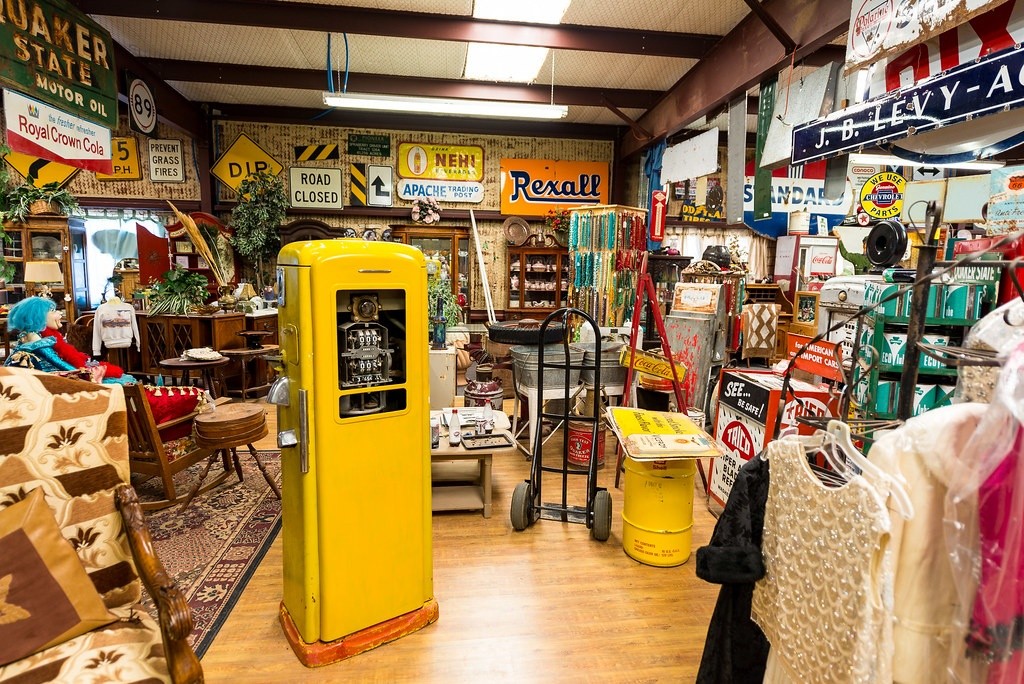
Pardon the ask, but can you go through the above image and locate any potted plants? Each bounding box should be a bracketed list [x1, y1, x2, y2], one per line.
[0, 176, 82, 280]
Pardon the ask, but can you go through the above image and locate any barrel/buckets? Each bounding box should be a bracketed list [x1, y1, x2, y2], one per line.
[564, 420, 606, 471]
[622, 454, 697, 567]
[788, 210, 810, 235]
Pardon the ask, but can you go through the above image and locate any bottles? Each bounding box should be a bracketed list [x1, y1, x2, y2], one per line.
[430, 417, 439, 449]
[129, 276, 162, 311]
[433, 297, 446, 343]
[217, 273, 273, 312]
[484, 399, 494, 433]
[535, 224, 547, 248]
[449, 409, 461, 447]
[510, 260, 570, 307]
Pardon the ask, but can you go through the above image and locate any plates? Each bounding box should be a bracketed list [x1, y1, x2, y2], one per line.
[503, 216, 531, 245]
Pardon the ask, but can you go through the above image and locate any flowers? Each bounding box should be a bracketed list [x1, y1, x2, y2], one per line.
[545, 209, 571, 233]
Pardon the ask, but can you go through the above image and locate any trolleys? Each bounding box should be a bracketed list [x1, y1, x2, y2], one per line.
[510, 306, 612, 540]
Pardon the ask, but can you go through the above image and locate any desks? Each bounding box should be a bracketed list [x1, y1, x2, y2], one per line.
[512, 384, 620, 459]
[429, 427, 492, 519]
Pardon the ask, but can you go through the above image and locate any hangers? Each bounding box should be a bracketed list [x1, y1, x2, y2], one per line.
[796, 386, 914, 518]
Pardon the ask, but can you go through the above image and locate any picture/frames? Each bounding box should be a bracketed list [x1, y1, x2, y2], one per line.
[793, 291, 821, 325]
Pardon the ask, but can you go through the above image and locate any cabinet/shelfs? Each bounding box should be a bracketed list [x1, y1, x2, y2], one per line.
[246, 319, 279, 386]
[505, 245, 569, 321]
[120, 316, 246, 401]
[844, 309, 990, 460]
[0, 218, 76, 334]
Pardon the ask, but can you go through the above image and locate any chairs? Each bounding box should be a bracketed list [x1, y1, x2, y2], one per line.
[0, 363, 204, 684]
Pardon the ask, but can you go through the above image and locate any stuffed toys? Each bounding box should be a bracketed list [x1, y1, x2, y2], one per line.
[8, 297, 163, 386]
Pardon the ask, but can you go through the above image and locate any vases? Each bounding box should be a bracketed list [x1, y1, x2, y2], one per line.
[555, 230, 569, 247]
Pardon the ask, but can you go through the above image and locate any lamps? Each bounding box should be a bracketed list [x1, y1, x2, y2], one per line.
[322, 34, 568, 120]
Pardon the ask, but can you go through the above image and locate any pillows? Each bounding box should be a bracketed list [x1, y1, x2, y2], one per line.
[0, 488, 122, 667]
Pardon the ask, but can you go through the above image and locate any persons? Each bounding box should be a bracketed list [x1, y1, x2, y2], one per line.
[833, 230, 874, 274]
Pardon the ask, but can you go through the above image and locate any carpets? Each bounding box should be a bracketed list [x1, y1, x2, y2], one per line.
[153, 449, 285, 658]
